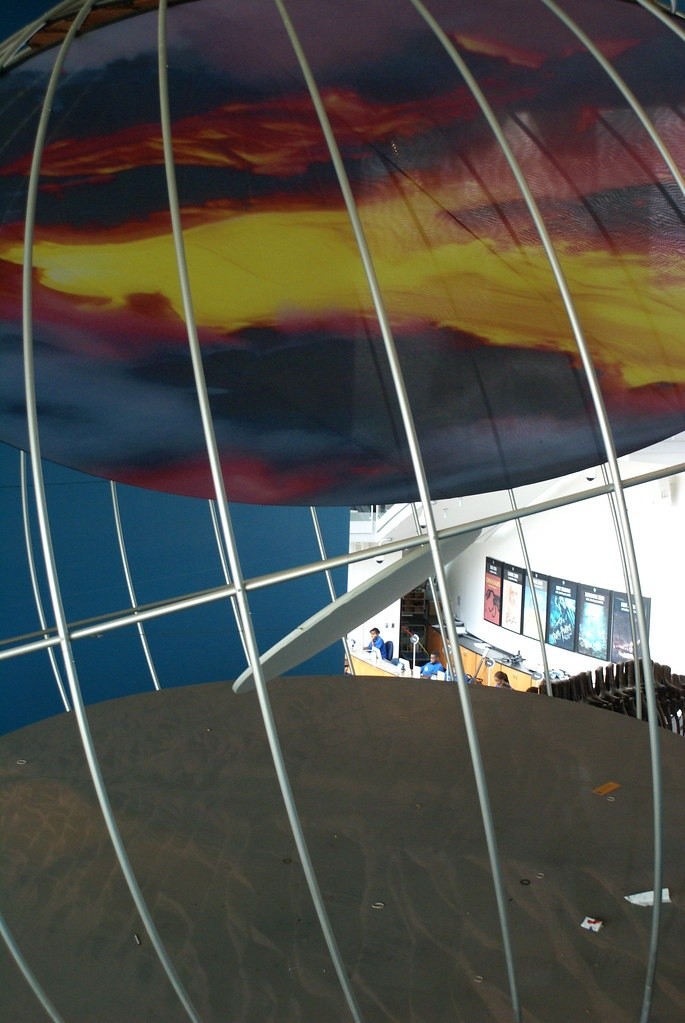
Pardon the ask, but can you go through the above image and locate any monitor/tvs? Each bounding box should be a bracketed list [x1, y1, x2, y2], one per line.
[399, 658, 410, 673]
[372, 647, 382, 660]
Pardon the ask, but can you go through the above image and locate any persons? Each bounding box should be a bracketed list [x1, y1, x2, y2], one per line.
[362, 627, 387, 660]
[420, 651, 443, 680]
[492, 670, 512, 689]
[444, 664, 468, 683]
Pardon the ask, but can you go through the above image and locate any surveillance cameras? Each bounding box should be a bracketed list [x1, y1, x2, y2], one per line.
[376, 557, 384, 563]
[585, 467, 596, 481]
[418, 516, 427, 529]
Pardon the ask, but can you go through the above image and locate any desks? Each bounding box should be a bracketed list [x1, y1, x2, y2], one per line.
[348, 647, 403, 677]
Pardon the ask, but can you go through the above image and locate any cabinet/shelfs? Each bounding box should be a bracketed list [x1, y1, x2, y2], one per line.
[426, 624, 531, 692]
